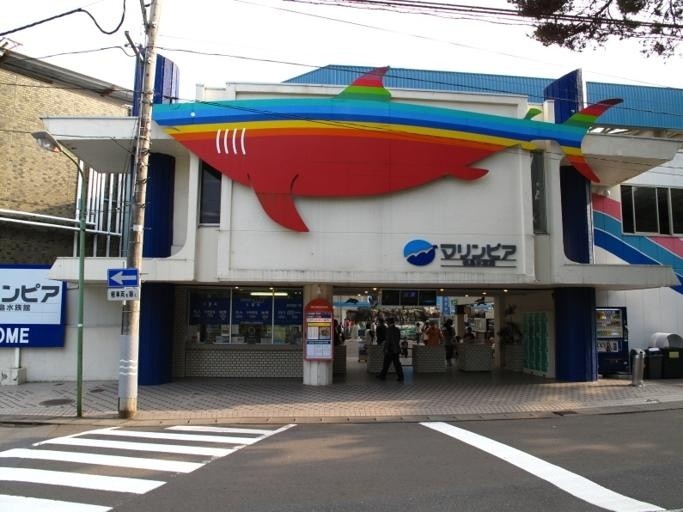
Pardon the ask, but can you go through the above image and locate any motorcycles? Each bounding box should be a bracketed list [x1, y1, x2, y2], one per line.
[399, 337, 408, 357]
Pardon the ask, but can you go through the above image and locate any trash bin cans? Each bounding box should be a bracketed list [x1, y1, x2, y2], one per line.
[645, 348, 664, 379]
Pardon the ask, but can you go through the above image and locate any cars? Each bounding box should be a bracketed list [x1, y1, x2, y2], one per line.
[400, 328, 416, 341]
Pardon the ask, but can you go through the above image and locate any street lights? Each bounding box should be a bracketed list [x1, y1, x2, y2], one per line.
[27, 128, 88, 419]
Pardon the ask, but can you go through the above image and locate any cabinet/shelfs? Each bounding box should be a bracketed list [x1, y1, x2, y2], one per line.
[520, 310, 556, 378]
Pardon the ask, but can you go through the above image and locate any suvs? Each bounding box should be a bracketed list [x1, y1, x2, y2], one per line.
[470, 318, 494, 339]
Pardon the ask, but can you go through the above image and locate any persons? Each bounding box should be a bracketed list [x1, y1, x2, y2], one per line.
[334, 318, 475, 381]
